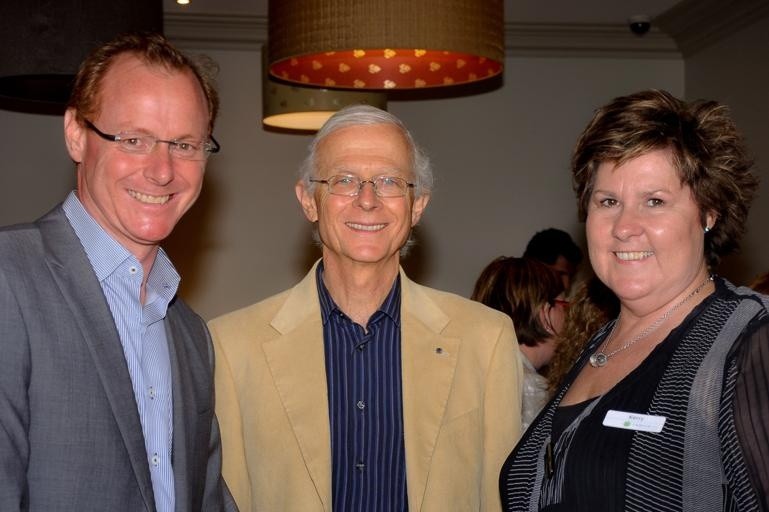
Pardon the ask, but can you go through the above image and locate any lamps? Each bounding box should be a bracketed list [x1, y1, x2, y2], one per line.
[261, 44, 387, 131]
[267, 0, 505, 89]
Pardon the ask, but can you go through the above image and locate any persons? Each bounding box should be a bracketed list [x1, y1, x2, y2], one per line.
[543, 269, 621, 400]
[202, 101, 525, 511]
[0, 28, 242, 511]
[497, 85, 768, 512]
[518, 226, 582, 296]
[466, 252, 571, 440]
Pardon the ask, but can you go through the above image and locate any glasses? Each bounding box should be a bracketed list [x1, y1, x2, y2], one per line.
[308, 172, 417, 199]
[82, 116, 222, 163]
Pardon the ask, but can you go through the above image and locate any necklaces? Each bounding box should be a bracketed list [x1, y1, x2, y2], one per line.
[588, 273, 715, 368]
